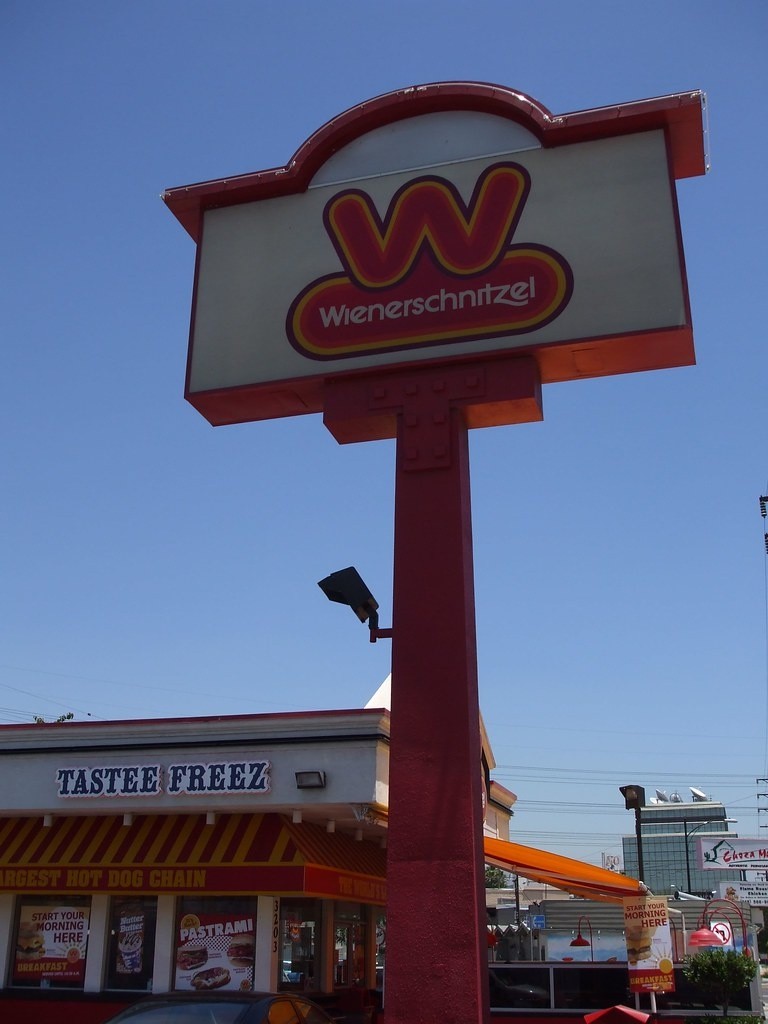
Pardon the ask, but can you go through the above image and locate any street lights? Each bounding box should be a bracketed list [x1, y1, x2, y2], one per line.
[618, 784, 647, 882]
[683, 817, 737, 894]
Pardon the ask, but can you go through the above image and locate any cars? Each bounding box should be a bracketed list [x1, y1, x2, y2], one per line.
[104, 991, 336, 1024]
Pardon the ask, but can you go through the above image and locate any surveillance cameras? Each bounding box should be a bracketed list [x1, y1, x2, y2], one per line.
[317, 567, 392, 643]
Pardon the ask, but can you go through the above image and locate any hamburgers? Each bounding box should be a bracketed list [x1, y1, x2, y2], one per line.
[17, 929, 46, 958]
[176, 945, 208, 970]
[626, 936, 652, 962]
[227, 933, 255, 967]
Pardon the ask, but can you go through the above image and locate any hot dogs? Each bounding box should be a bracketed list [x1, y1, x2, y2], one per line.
[189, 966, 232, 990]
